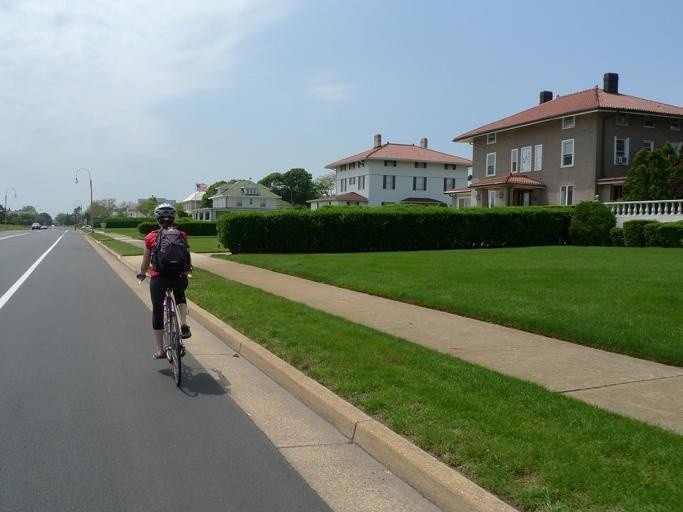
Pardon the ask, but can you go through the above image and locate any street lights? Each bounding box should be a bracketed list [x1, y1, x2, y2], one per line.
[74, 167, 94, 233]
[3, 188, 17, 231]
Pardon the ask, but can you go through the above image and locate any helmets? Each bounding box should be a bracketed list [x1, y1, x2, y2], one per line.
[154, 203, 176, 221]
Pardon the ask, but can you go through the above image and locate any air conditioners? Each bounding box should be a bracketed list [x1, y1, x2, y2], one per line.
[619, 156, 627, 163]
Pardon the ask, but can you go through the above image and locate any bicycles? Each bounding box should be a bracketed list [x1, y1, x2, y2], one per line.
[136, 267, 195, 388]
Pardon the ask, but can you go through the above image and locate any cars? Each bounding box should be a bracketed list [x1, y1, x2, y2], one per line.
[80, 224, 93, 231]
[31, 222, 41, 230]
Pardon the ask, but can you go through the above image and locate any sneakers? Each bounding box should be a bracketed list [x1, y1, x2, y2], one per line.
[180, 324, 191, 337]
[154, 350, 165, 358]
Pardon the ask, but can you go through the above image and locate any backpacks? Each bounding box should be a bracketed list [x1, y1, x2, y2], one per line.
[150, 226, 191, 272]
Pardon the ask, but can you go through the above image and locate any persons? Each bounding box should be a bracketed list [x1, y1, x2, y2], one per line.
[133, 202, 192, 360]
